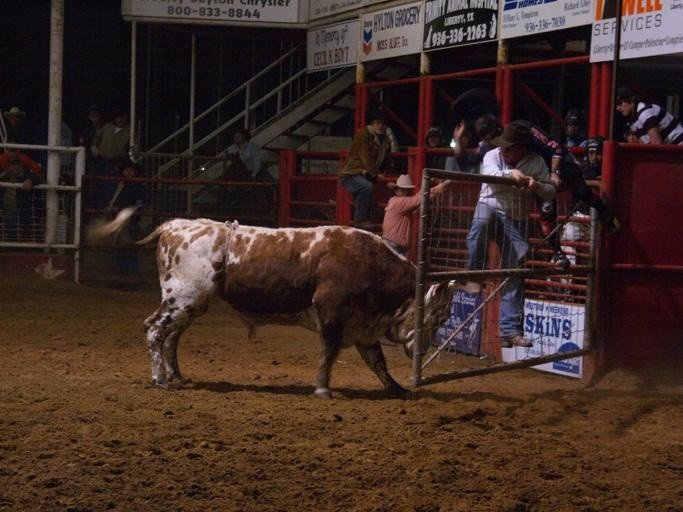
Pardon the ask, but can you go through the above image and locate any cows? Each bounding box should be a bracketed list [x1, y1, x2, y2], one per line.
[102, 204, 459, 403]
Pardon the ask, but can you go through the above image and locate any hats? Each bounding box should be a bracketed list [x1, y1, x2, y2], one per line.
[567, 111, 582, 125]
[425, 127, 443, 140]
[589, 136, 603, 152]
[491, 123, 528, 148]
[4, 107, 25, 117]
[388, 175, 415, 189]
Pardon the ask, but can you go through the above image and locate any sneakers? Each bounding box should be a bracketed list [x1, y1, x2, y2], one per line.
[499, 335, 532, 348]
[550, 252, 570, 265]
[351, 221, 375, 232]
[468, 280, 482, 294]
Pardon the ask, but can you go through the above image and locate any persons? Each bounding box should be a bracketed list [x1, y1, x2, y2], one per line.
[382, 172, 451, 256]
[464, 120, 556, 350]
[420, 83, 682, 182]
[0, 102, 146, 291]
[226, 124, 274, 228]
[336, 105, 392, 232]
[462, 111, 620, 265]
